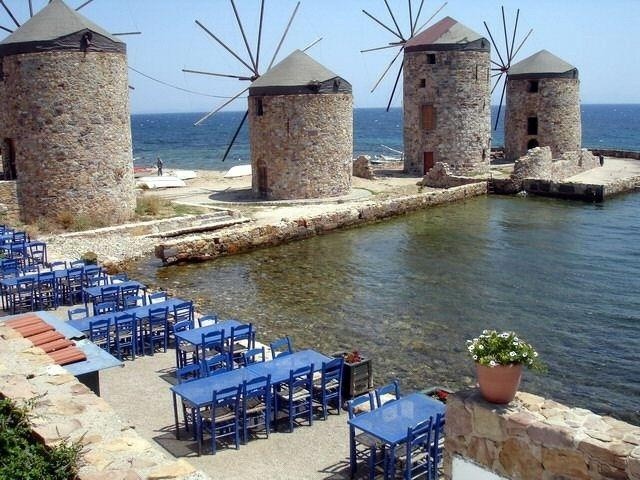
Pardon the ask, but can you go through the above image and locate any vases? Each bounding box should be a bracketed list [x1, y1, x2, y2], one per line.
[475, 363, 527, 403]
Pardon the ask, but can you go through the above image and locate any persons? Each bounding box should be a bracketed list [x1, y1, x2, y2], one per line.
[157, 156, 163, 177]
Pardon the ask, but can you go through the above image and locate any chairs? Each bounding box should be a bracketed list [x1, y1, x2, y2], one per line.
[169, 336, 345, 457]
[1, 224, 194, 361]
[345, 380, 446, 479]
[171, 314, 256, 378]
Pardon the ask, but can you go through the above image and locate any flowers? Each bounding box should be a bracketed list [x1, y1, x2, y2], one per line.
[465, 330, 537, 369]
